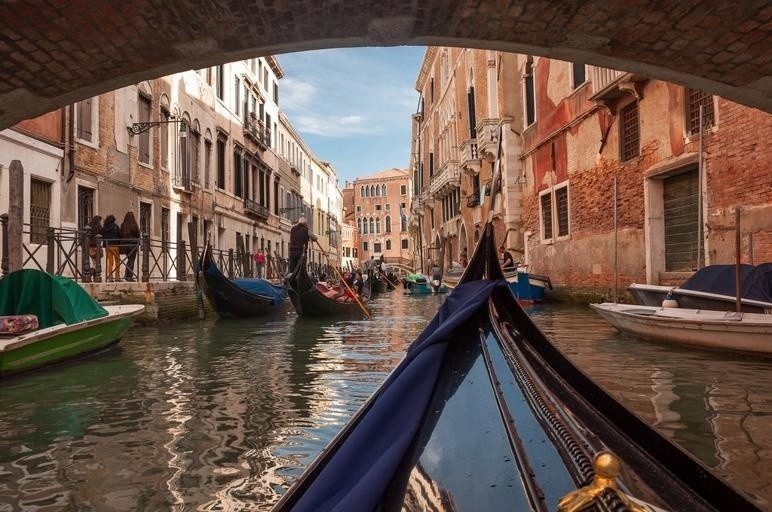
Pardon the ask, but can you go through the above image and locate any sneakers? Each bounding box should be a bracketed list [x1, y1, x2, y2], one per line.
[109, 277, 136, 282]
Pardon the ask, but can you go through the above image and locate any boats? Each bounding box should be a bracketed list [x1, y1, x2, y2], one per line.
[400, 272, 448, 294]
[269, 126, 767, 512]
[588, 262, 772, 358]
[504, 270, 555, 309]
[0, 269, 146, 379]
[198, 239, 398, 319]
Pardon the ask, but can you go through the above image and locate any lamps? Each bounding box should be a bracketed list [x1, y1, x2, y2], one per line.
[127, 118, 188, 138]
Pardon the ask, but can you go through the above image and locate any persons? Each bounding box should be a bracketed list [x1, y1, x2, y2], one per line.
[381, 260, 386, 276]
[429, 264, 440, 280]
[308, 270, 363, 299]
[498, 245, 514, 272]
[459, 248, 467, 265]
[289, 217, 318, 273]
[102, 214, 120, 282]
[255, 248, 266, 277]
[364, 256, 377, 280]
[121, 211, 140, 282]
[84, 216, 103, 278]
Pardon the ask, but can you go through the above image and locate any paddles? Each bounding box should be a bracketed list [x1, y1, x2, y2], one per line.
[316, 239, 373, 318]
[621, 309, 743, 321]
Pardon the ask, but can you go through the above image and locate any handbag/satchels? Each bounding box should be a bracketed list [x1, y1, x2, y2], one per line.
[89, 247, 102, 258]
